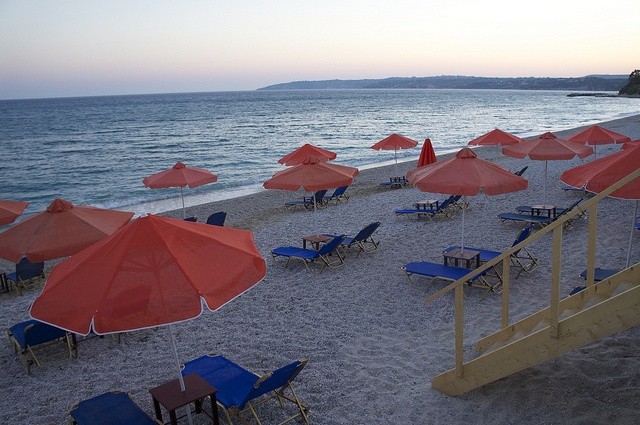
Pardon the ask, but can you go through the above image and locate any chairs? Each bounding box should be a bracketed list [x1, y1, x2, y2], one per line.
[8, 312, 82, 376]
[563, 184, 588, 195]
[181, 352, 309, 424]
[329, 185, 350, 206]
[272, 232, 344, 275]
[377, 177, 408, 189]
[401, 260, 504, 294]
[515, 198, 590, 210]
[569, 284, 586, 298]
[7, 252, 49, 298]
[442, 225, 539, 283]
[412, 193, 469, 207]
[582, 268, 620, 282]
[320, 222, 382, 259]
[285, 188, 328, 213]
[204, 210, 228, 227]
[183, 216, 198, 222]
[394, 196, 453, 221]
[513, 164, 530, 177]
[70, 391, 159, 425]
[496, 213, 571, 229]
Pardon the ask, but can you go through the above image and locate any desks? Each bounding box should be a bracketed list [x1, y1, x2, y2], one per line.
[525, 205, 558, 219]
[416, 198, 440, 219]
[302, 235, 332, 251]
[443, 249, 483, 268]
[148, 372, 218, 424]
[389, 176, 406, 185]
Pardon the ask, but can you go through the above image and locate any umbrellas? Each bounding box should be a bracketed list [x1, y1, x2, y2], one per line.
[371, 134, 419, 173]
[417, 138, 437, 168]
[501, 129, 593, 205]
[407, 148, 526, 254]
[23, 214, 272, 424]
[568, 126, 631, 159]
[277, 144, 339, 168]
[468, 127, 527, 157]
[261, 164, 359, 237]
[0, 199, 140, 263]
[2, 200, 28, 227]
[142, 162, 217, 217]
[560, 137, 639, 270]
